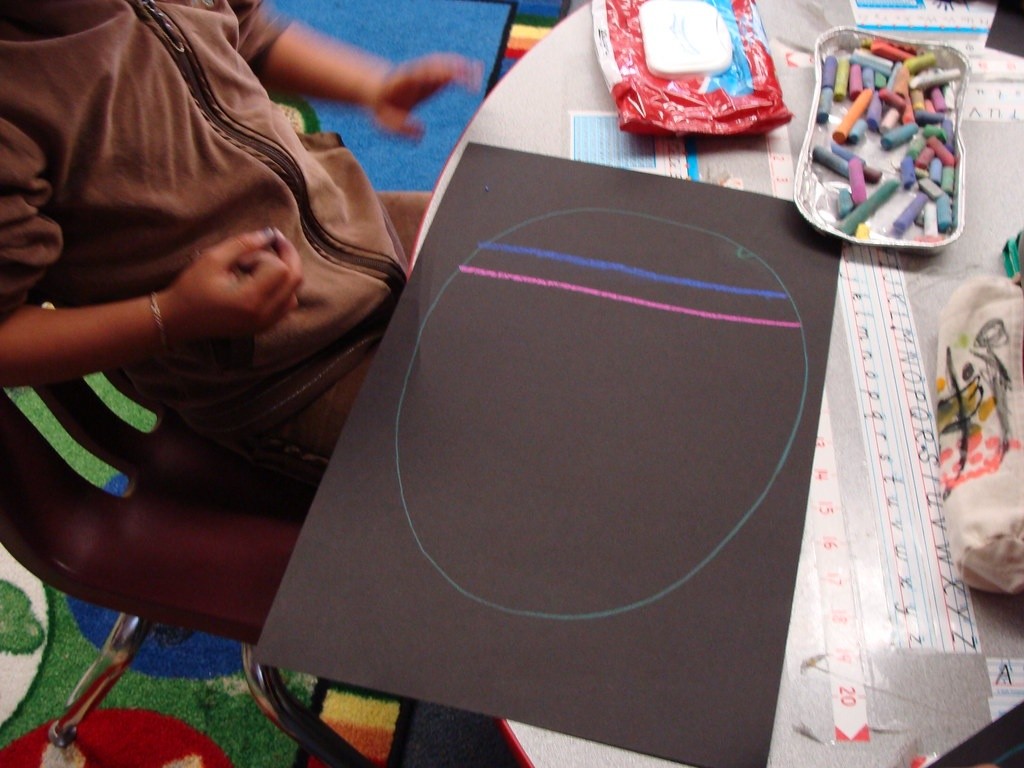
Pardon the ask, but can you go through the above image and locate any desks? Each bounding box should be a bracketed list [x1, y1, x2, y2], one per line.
[406, 0, 1024, 768]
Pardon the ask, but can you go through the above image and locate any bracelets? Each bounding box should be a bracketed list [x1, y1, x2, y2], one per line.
[150, 291, 169, 356]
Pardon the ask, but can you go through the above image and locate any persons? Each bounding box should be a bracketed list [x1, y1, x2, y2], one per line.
[0, 0, 486, 519]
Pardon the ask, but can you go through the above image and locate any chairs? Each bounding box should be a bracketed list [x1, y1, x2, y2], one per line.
[0, 190, 445, 768]
